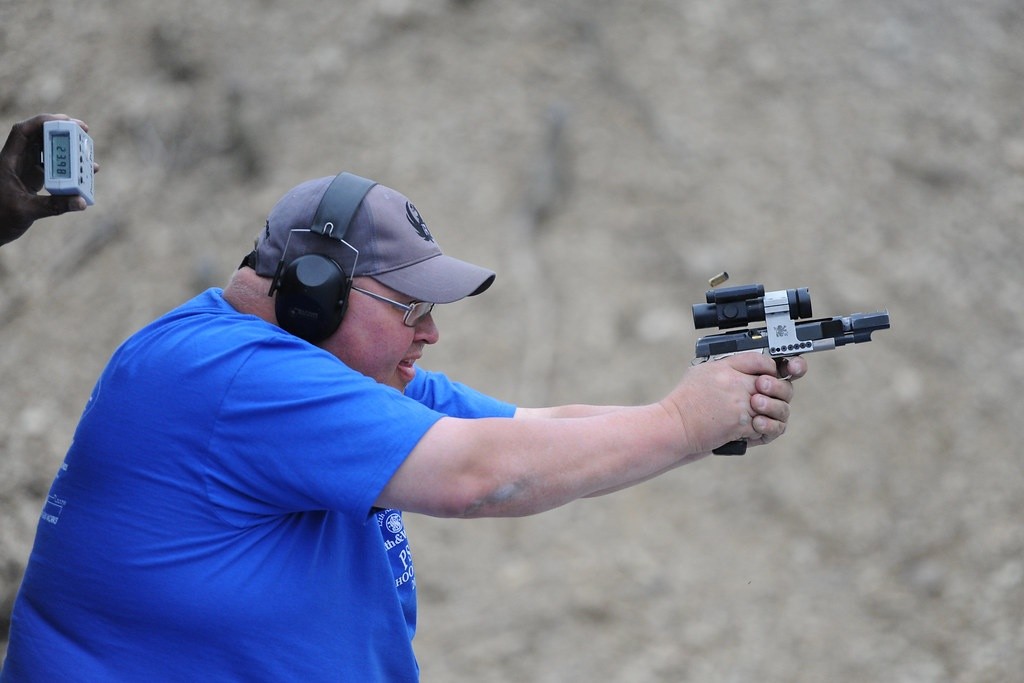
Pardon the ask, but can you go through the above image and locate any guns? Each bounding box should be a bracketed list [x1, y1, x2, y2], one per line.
[690, 285, 891, 457]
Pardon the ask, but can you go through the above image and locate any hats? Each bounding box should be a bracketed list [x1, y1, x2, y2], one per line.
[238, 173, 497, 305]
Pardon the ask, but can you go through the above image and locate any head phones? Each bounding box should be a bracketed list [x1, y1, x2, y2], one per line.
[269, 172, 380, 341]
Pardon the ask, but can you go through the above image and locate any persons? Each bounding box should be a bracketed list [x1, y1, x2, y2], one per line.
[0, 115, 99, 249]
[0, 174, 809, 683]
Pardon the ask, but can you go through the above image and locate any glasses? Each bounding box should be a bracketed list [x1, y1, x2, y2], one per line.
[351, 286, 435, 326]
